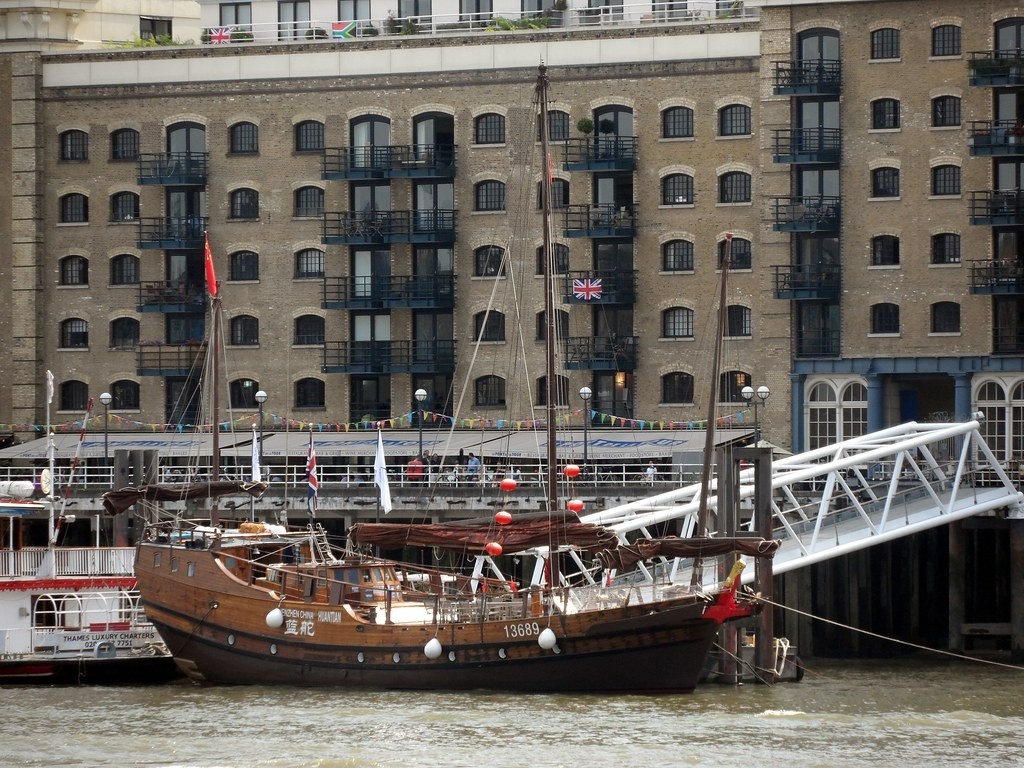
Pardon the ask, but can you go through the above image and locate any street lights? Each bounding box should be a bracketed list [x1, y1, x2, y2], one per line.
[255, 390, 268, 474]
[578, 387, 594, 487]
[414, 388, 429, 487]
[741, 384, 771, 448]
[98, 392, 113, 482]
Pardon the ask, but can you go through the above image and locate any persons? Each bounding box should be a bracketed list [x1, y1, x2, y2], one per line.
[341, 450, 540, 489]
[164, 465, 284, 482]
[647, 460, 657, 482]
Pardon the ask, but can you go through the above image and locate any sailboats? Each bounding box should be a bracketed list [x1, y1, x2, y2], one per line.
[104, 51, 782, 693]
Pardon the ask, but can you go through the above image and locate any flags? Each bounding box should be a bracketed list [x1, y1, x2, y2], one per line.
[374, 430, 393, 516]
[332, 21, 356, 39]
[252, 430, 261, 483]
[205, 235, 218, 296]
[210, 27, 230, 43]
[305, 428, 318, 518]
[573, 278, 603, 301]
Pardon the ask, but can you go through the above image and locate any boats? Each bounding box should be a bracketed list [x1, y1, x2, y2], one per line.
[1, 365, 455, 689]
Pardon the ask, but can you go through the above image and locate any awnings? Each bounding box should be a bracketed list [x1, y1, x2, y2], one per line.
[0, 428, 762, 460]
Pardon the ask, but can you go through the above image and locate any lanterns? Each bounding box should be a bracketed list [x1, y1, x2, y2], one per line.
[495, 511, 512, 525]
[501, 478, 517, 492]
[486, 542, 503, 557]
[569, 499, 584, 513]
[564, 464, 580, 478]
